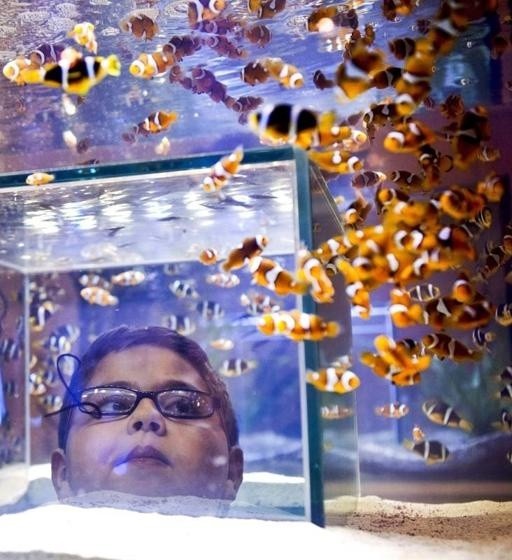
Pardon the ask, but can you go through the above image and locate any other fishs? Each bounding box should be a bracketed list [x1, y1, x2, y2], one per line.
[0, 0, 512, 465]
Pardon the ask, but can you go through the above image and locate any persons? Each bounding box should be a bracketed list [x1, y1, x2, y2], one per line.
[49, 325, 247, 518]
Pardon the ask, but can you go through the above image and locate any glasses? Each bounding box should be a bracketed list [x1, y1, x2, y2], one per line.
[77, 386, 221, 420]
